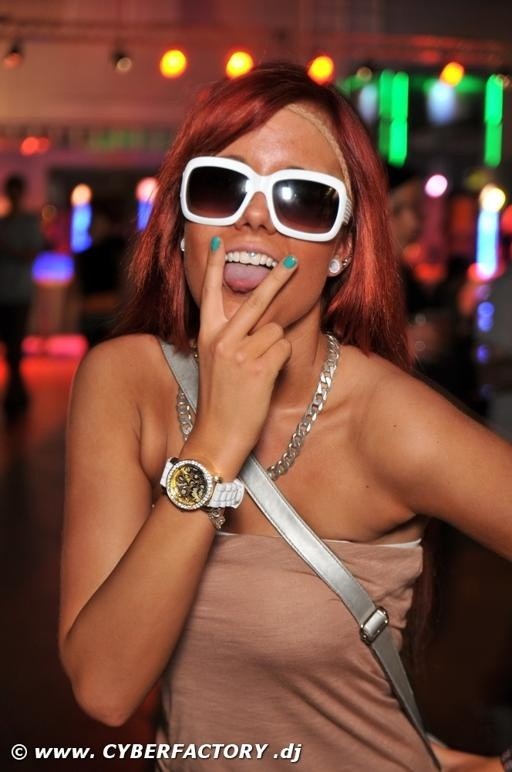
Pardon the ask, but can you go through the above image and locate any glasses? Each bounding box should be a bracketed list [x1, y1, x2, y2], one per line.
[179, 156, 353, 242]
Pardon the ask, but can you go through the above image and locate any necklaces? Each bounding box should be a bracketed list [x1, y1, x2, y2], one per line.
[176, 333, 340, 481]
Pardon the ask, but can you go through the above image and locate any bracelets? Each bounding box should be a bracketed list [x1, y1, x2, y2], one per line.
[205, 507, 226, 530]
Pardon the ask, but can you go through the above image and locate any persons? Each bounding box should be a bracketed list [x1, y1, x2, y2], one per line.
[68, 203, 128, 349]
[0, 173, 46, 423]
[56, 53, 512, 771]
[399, 232, 511, 447]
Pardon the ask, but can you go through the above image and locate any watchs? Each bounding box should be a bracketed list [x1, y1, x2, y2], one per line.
[158, 455, 245, 511]
